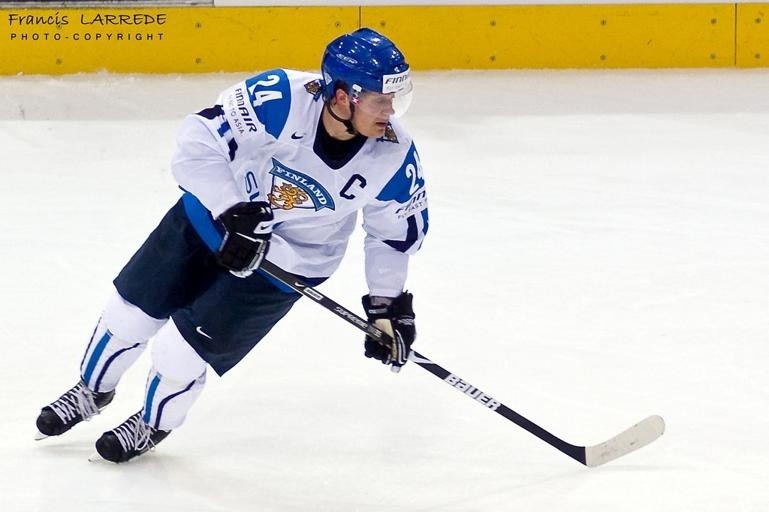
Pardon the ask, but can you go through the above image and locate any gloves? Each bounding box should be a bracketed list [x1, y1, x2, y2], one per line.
[361, 291, 416, 373]
[218, 202, 273, 277]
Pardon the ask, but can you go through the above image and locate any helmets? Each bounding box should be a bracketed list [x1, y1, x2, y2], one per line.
[321, 27, 411, 99]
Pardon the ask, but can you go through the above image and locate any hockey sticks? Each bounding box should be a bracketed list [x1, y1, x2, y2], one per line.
[259, 260, 665, 467]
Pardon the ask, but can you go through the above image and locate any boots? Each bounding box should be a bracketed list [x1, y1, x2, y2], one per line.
[96, 406, 172, 463]
[36, 381, 116, 435]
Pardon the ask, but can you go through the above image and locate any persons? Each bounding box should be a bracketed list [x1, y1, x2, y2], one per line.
[36, 28, 429, 464]
